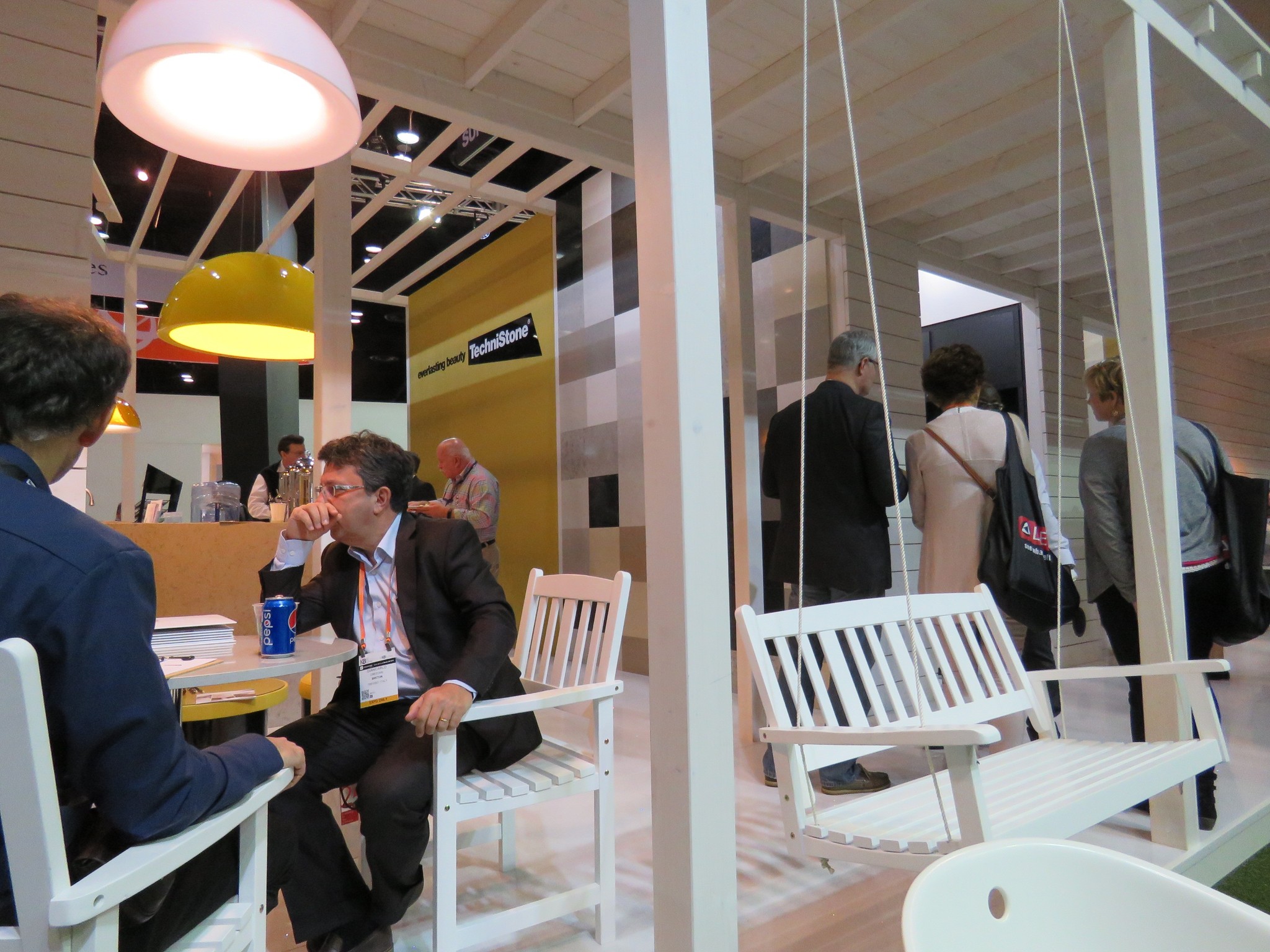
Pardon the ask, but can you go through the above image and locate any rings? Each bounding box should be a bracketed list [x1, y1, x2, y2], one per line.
[439, 718, 450, 722]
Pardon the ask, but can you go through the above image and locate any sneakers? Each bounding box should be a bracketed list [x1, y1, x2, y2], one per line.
[822, 761, 891, 796]
[764, 768, 782, 787]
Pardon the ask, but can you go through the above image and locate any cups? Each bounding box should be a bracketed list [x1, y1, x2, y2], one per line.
[269, 503, 287, 521]
[251, 603, 300, 645]
[163, 512, 182, 523]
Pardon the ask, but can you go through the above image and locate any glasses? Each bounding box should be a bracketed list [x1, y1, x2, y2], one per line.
[975, 398, 1005, 411]
[313, 483, 379, 501]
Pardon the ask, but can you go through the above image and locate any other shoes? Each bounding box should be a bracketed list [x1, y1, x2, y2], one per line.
[360, 863, 430, 928]
[1028, 720, 1057, 742]
[1188, 769, 1219, 833]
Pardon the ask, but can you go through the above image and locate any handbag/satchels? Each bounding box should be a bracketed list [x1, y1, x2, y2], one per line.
[1182, 415, 1270, 652]
[979, 411, 1081, 629]
[67, 802, 181, 933]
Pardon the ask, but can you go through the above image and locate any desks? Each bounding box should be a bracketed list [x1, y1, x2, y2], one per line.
[164, 632, 357, 745]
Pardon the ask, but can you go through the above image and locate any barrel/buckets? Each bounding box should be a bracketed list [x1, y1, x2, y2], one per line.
[279, 449, 314, 517]
[191, 481, 240, 523]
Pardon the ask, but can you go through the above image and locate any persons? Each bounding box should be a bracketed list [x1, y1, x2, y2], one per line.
[259, 434, 542, 952]
[900, 343, 1078, 740]
[1, 291, 396, 950]
[247, 435, 307, 521]
[1082, 353, 1231, 826]
[762, 332, 908, 792]
[407, 437, 500, 580]
[404, 451, 437, 503]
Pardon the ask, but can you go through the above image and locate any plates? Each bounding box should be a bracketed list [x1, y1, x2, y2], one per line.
[407, 505, 429, 509]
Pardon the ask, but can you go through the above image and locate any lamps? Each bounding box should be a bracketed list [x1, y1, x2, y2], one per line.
[151, 169, 359, 367]
[94, 255, 142, 436]
[98, 2, 368, 181]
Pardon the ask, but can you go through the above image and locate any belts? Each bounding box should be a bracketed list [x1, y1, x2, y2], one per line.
[477, 539, 497, 550]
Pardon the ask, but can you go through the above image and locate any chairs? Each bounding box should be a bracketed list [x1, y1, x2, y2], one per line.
[357, 559, 638, 952]
[3, 636, 300, 952]
[897, 840, 1269, 952]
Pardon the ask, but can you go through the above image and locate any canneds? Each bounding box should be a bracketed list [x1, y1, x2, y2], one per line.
[256, 593, 300, 658]
